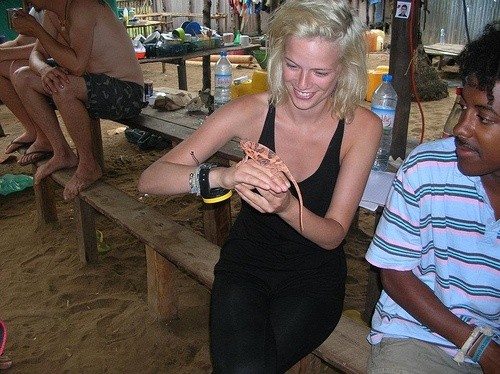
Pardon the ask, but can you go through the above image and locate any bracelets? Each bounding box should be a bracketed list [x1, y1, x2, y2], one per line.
[187, 150, 233, 204]
[452, 324, 495, 367]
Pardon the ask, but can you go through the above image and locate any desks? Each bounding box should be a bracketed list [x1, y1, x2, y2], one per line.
[425, 42, 467, 70]
[76, 86, 403, 327]
[134, 13, 161, 38]
[161, 12, 228, 36]
[127, 20, 173, 73]
[138, 42, 261, 95]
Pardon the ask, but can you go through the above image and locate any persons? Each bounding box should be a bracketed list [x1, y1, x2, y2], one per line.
[365, 19, 500, 374]
[0, 0, 150, 202]
[398, 4, 407, 17]
[137, 0, 382, 374]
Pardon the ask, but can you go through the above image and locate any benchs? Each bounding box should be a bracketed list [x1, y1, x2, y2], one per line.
[16, 142, 375, 374]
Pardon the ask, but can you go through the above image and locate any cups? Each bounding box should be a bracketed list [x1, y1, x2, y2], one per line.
[6, 7, 23, 30]
[240, 35, 252, 46]
[223, 33, 234, 43]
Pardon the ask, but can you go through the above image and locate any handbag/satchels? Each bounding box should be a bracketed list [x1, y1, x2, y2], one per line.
[152, 92, 192, 112]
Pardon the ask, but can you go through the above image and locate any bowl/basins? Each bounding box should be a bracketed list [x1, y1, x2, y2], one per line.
[137, 53, 146, 59]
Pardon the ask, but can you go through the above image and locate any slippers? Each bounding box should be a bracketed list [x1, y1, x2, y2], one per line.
[6, 137, 33, 153]
[20, 150, 53, 166]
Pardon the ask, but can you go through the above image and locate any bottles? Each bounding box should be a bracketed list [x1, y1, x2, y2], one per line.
[234, 75, 249, 85]
[442, 87, 463, 137]
[440, 28, 445, 46]
[149, 44, 184, 59]
[129, 10, 134, 19]
[123, 7, 129, 25]
[371, 74, 398, 172]
[213, 51, 233, 111]
